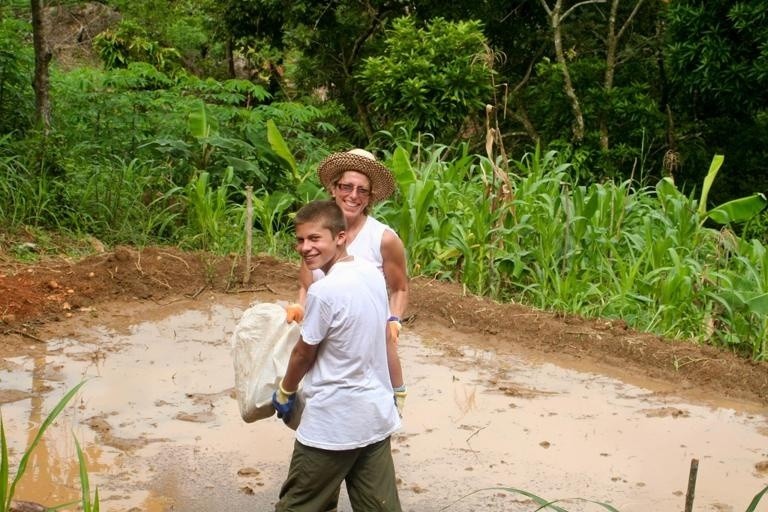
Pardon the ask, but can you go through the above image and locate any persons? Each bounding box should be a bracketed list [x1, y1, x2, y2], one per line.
[275, 200, 408, 512]
[285, 148, 408, 343]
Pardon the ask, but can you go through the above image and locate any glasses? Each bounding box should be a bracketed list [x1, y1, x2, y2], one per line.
[335, 183, 371, 197]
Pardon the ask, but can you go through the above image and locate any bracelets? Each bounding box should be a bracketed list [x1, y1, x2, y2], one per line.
[387, 316, 402, 330]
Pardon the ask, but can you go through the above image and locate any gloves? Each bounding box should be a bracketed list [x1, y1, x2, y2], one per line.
[272, 377, 296, 423]
[394, 385, 408, 414]
[285, 304, 305, 324]
[388, 316, 402, 343]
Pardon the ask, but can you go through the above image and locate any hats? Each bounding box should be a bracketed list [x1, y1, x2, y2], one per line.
[317, 148, 395, 205]
